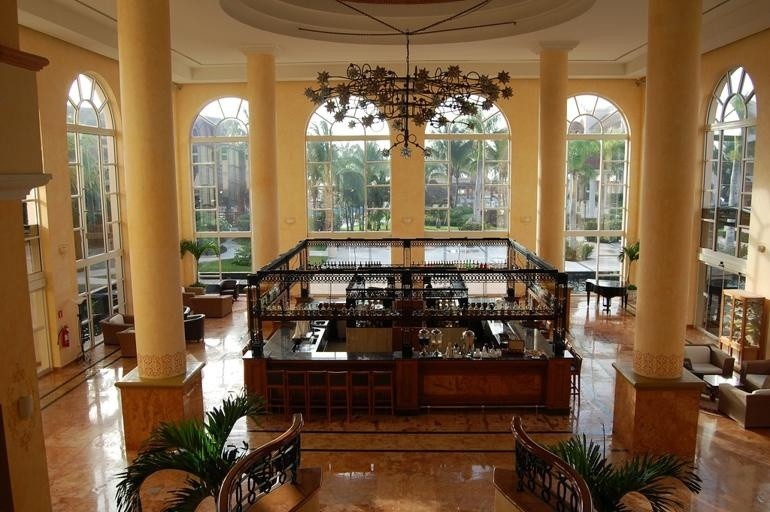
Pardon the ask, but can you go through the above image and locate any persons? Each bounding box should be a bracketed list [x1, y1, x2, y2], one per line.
[504, 288, 519, 305]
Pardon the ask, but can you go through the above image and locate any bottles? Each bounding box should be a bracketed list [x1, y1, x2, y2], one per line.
[250, 258, 567, 363]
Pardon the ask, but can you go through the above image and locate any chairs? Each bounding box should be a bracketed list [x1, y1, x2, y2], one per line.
[99, 313, 133, 345]
[740, 359, 770, 390]
[684, 344, 735, 376]
[718, 383, 770, 429]
[184, 314, 204, 343]
[219, 281, 239, 301]
[182, 287, 205, 305]
[565, 338, 582, 406]
[183, 306, 191, 319]
[262, 369, 394, 424]
[115, 327, 136, 358]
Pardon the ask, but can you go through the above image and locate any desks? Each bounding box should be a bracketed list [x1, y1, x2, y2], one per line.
[190, 294, 233, 318]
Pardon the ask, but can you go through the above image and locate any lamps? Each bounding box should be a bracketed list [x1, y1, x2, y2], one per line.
[303, 35, 514, 160]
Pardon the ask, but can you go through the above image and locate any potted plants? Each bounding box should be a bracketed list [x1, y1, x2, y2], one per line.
[618, 242, 639, 295]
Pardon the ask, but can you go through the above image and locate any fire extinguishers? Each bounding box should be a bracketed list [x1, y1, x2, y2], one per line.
[57, 325, 70, 347]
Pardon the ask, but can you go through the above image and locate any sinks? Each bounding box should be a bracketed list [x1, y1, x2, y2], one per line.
[303, 338, 317, 345]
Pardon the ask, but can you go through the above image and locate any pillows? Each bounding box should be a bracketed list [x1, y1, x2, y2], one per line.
[109, 314, 124, 324]
